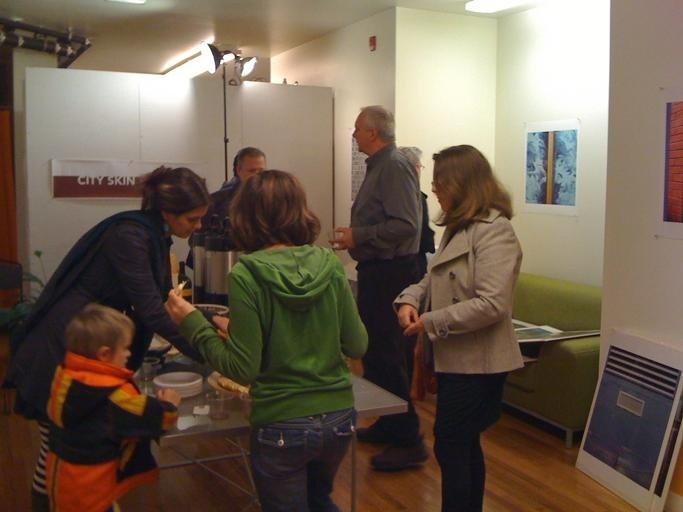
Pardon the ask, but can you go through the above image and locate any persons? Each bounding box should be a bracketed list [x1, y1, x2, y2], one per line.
[326, 105, 431, 469]
[167, 169, 367, 512]
[398, 138, 436, 272]
[391, 145, 523, 511]
[185, 148, 265, 263]
[45, 302, 181, 512]
[7, 159, 231, 509]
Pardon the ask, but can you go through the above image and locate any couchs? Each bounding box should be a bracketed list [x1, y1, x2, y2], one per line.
[502, 270, 602, 447]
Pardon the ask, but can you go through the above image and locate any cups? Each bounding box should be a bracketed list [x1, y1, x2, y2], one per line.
[206, 391, 234, 419]
[239, 392, 252, 420]
[327, 231, 344, 248]
[141, 358, 159, 381]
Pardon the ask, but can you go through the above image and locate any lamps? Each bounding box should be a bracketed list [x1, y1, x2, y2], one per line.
[198, 38, 257, 79]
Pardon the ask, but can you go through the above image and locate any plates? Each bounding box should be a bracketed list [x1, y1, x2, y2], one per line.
[152, 371, 204, 398]
[208, 371, 246, 394]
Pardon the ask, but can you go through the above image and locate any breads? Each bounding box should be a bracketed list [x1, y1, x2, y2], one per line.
[215, 376, 248, 395]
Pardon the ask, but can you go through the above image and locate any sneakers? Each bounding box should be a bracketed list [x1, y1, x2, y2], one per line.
[370, 443, 430, 472]
[355, 420, 392, 443]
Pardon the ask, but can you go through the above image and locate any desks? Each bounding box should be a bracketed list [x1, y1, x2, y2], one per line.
[132, 328, 409, 512]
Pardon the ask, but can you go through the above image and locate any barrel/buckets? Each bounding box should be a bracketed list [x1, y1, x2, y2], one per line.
[191, 215, 245, 305]
